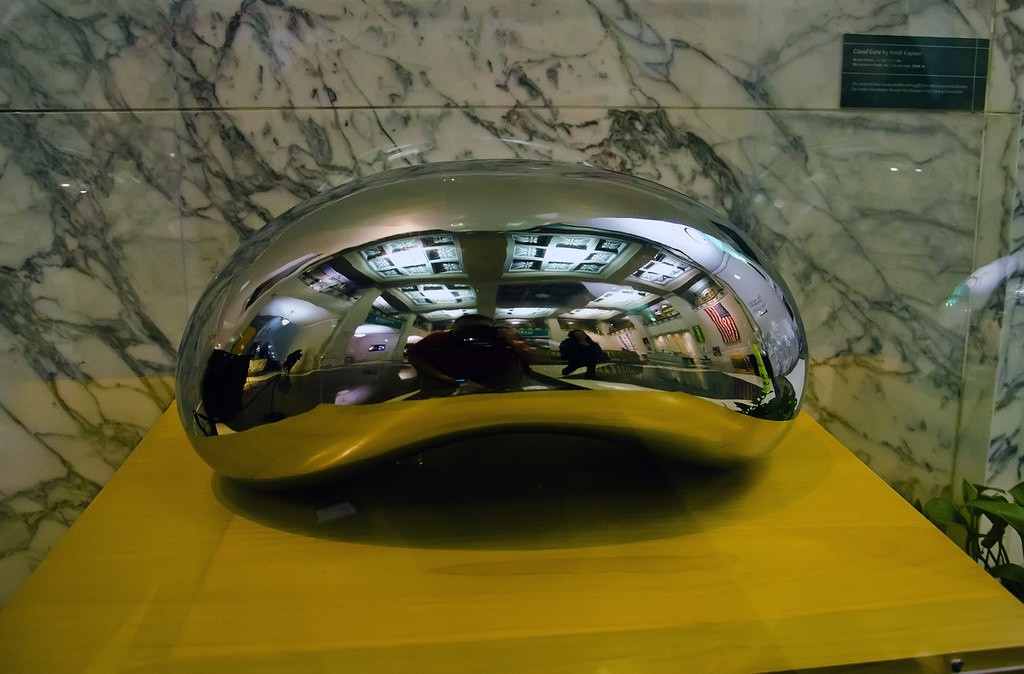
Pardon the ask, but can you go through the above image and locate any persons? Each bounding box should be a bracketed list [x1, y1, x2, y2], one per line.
[405, 314, 532, 397]
[258, 341, 271, 360]
[282, 349, 303, 373]
[558, 330, 611, 379]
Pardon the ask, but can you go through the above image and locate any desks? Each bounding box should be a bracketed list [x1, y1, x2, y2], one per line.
[0, 401, 1024, 674]
